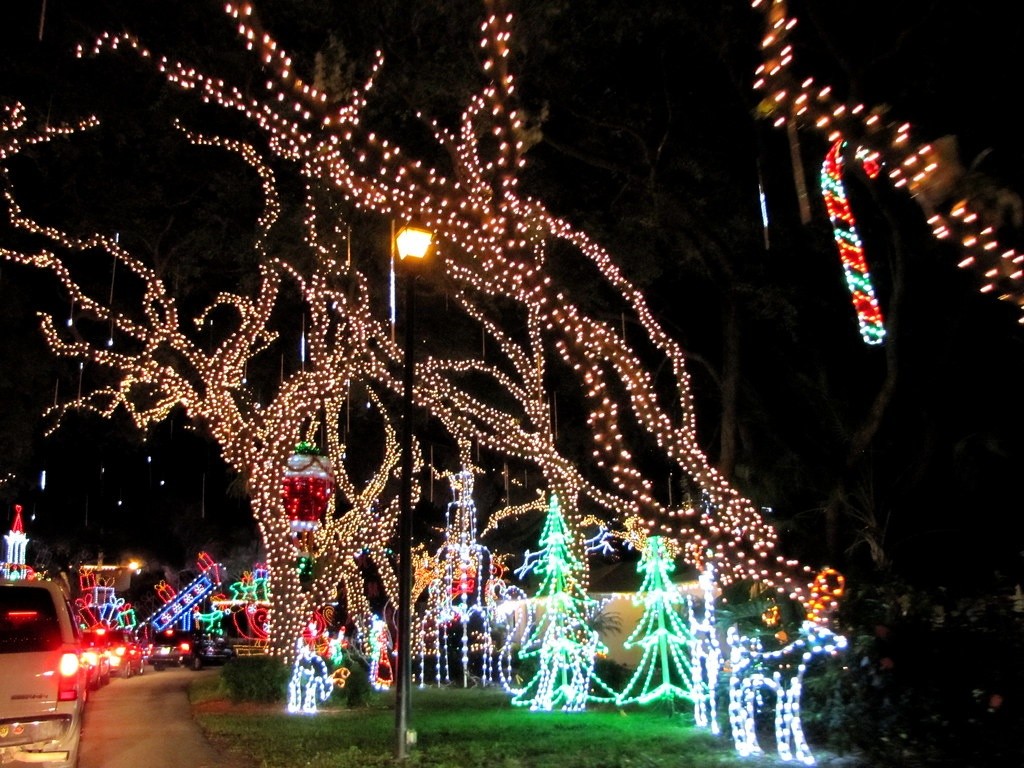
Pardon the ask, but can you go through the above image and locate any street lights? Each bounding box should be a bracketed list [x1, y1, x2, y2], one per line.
[384, 202, 439, 755]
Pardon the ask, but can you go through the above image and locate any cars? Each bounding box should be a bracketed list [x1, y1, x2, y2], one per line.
[0, 576, 235, 767]
